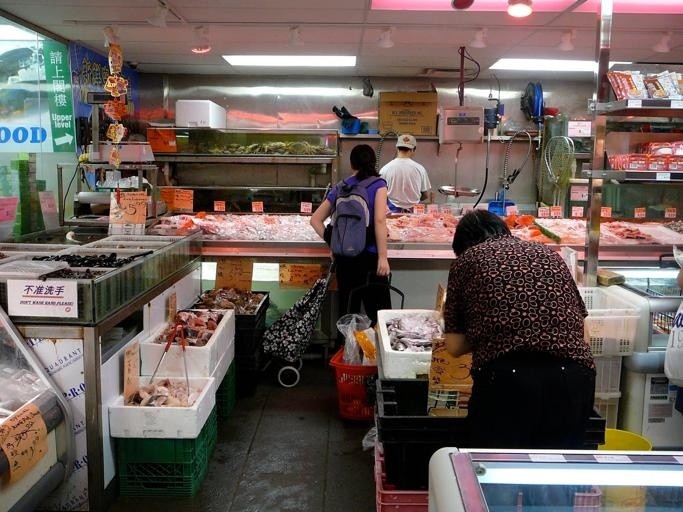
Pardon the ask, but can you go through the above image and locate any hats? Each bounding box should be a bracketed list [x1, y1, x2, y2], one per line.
[396, 135, 416, 149]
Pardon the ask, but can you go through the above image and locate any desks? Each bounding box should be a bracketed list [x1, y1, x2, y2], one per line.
[15, 256, 202, 511]
[56, 163, 157, 227]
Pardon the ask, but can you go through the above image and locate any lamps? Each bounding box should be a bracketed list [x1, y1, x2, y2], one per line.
[557, 32, 573, 51]
[377, 30, 393, 47]
[286, 28, 304, 48]
[145, 5, 167, 27]
[654, 34, 670, 53]
[470, 31, 485, 47]
[191, 29, 211, 53]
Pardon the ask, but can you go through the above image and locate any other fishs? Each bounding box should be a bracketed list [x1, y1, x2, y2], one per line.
[387, 315, 444, 351]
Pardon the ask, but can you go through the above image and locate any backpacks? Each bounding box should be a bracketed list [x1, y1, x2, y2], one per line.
[330, 176, 387, 256]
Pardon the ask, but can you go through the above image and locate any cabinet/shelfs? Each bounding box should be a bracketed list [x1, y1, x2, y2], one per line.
[146, 128, 339, 215]
[581, 0, 683, 286]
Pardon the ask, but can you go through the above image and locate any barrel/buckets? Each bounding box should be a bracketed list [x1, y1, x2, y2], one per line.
[595, 429, 653, 512]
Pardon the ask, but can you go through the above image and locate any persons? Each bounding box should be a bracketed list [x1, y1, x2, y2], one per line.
[311, 143, 392, 354]
[442, 208, 598, 450]
[377, 134, 432, 214]
[675, 265, 683, 419]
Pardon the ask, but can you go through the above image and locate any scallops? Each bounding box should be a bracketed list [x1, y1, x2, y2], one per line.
[128, 378, 201, 407]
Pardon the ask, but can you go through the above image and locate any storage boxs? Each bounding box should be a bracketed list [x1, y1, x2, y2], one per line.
[379, 92, 438, 136]
[176, 100, 228, 128]
[107, 289, 270, 438]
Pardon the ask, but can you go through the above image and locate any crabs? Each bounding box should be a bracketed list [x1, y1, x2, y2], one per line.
[193, 287, 264, 315]
[190, 141, 336, 155]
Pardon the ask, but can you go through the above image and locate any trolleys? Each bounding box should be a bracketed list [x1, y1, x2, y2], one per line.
[255, 267, 335, 389]
[325, 272, 406, 424]
